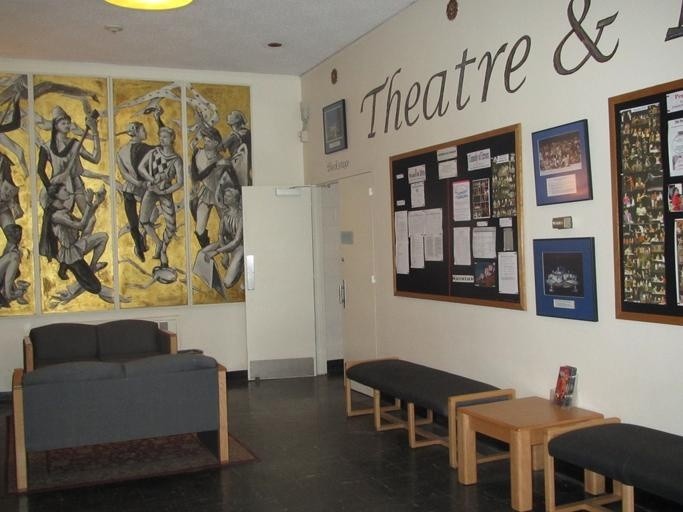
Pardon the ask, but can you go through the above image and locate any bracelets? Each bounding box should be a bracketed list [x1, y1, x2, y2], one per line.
[140, 181, 144, 189]
[214, 249, 219, 254]
[163, 189, 167, 195]
[93, 131, 98, 136]
[85, 201, 92, 207]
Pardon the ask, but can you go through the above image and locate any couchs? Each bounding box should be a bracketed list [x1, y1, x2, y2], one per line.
[22, 318, 177, 372]
[11, 354, 229, 493]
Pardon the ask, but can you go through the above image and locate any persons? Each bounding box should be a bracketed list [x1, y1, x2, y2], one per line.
[37, 105, 101, 238]
[219, 109, 252, 186]
[204, 187, 243, 288]
[139, 126, 183, 268]
[554, 367, 568, 400]
[116, 104, 165, 262]
[39, 183, 109, 294]
[491, 104, 682, 305]
[190, 127, 242, 248]
[0, 131, 31, 308]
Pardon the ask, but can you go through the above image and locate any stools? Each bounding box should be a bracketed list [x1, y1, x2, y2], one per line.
[455, 396, 603, 511]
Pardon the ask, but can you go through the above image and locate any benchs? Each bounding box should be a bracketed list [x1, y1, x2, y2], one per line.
[344, 357, 514, 468]
[542, 417, 682, 512]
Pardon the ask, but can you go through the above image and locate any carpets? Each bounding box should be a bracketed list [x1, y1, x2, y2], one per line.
[7, 415, 255, 495]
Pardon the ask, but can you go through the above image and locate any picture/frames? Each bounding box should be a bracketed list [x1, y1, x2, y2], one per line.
[533, 237, 599, 322]
[608, 79, 683, 326]
[322, 100, 348, 155]
[531, 120, 594, 208]
[388, 122, 527, 310]
